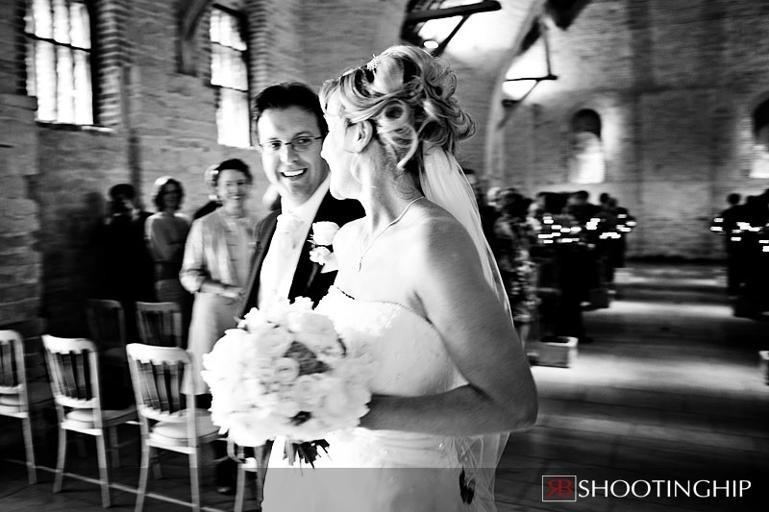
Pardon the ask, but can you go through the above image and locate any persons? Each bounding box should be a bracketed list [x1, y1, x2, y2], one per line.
[258, 44, 538, 512]
[235, 81, 362, 330]
[458, 164, 632, 362]
[78, 159, 261, 496]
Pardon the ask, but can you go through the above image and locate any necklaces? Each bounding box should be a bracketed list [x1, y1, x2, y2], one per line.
[356, 196, 426, 273]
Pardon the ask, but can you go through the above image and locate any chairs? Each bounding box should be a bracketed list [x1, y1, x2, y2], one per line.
[134, 301, 183, 348]
[0, 329, 86, 485]
[80, 300, 125, 363]
[39, 333, 163, 508]
[125, 343, 244, 512]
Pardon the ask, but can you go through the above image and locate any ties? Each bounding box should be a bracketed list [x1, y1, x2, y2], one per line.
[277, 217, 298, 251]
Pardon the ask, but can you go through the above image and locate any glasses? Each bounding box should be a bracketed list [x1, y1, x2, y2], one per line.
[255, 136, 322, 152]
